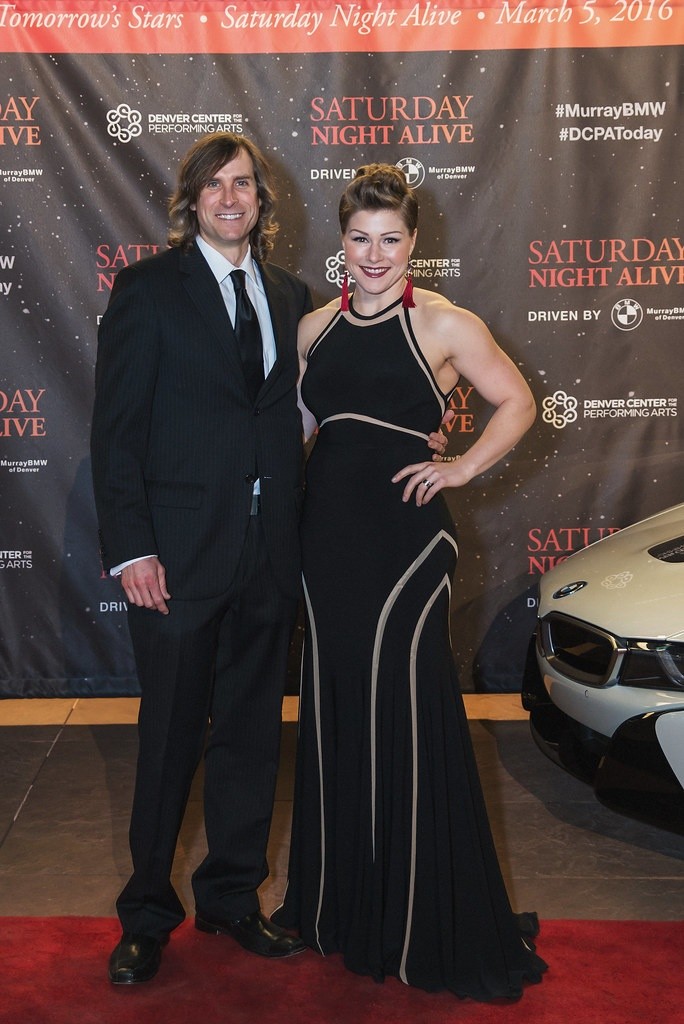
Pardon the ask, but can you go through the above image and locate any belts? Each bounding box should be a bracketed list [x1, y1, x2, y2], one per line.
[250, 495, 261, 516]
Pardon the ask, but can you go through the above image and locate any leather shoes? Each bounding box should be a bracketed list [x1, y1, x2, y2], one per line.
[107, 930, 162, 986]
[195, 912, 306, 958]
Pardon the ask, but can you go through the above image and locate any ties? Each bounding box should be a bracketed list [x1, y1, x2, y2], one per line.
[230, 270, 264, 403]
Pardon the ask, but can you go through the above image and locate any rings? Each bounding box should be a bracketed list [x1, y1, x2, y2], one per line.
[423, 479, 432, 488]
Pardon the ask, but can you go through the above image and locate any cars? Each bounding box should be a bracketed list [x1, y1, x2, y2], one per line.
[522, 500, 684, 811]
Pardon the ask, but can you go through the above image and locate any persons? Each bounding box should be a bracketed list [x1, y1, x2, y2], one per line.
[90, 133, 455, 985]
[270, 164, 550, 1004]
